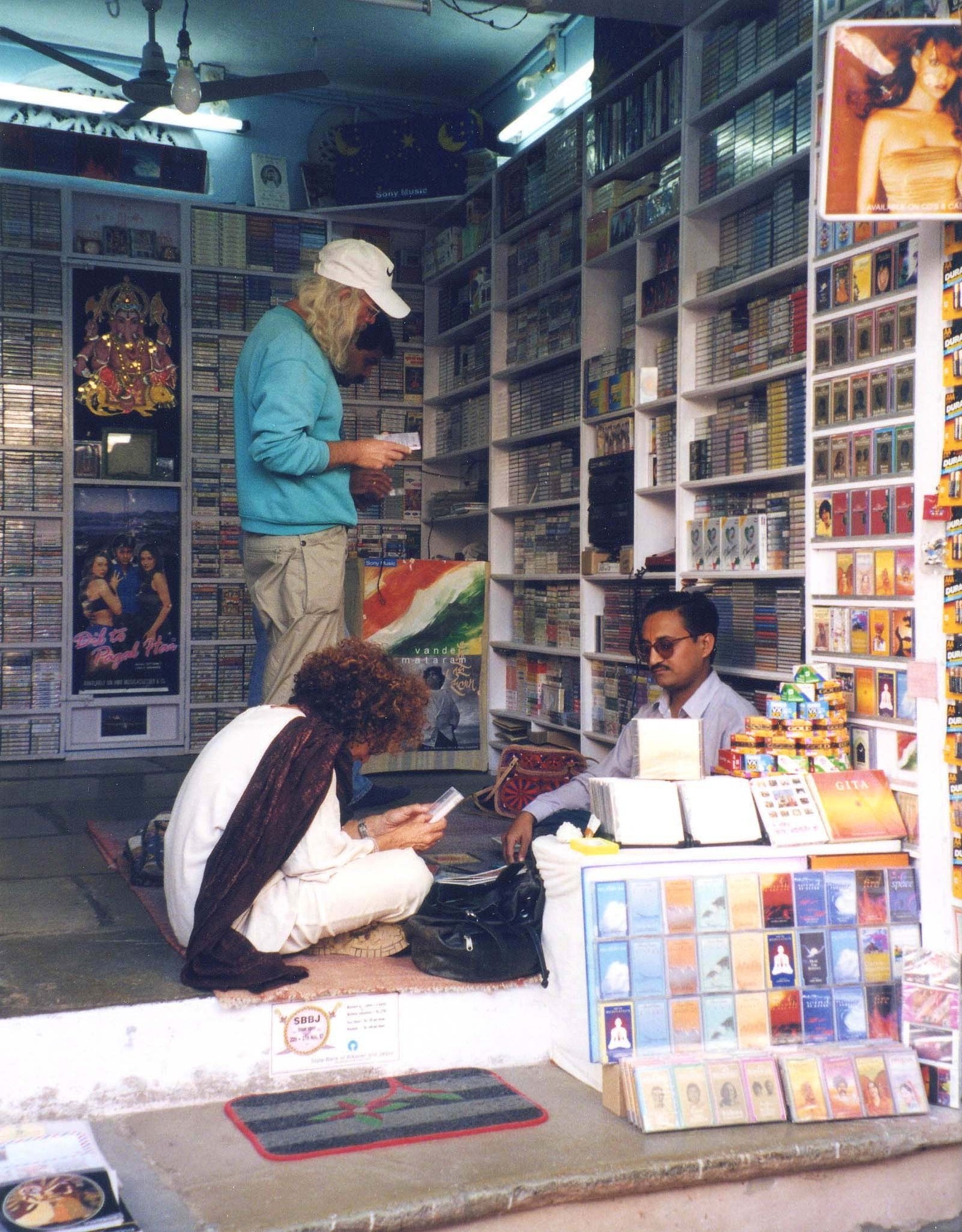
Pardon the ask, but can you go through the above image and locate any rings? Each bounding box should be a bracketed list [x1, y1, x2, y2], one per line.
[437, 839, 440, 843]
[372, 481, 377, 485]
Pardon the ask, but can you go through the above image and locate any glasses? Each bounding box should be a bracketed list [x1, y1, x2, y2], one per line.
[635, 633, 705, 663]
[358, 296, 381, 320]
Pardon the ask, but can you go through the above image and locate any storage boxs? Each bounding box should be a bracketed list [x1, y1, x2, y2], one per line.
[0, 0, 959, 1134]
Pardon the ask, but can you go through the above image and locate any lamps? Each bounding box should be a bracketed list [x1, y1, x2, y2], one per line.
[0, 81, 251, 135]
[359, 0, 432, 16]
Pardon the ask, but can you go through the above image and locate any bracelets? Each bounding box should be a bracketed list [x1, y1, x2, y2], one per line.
[365, 835, 379, 854]
[357, 817, 368, 839]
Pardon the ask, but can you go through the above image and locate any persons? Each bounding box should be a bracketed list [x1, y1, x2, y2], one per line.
[162, 636, 447, 957]
[816, 500, 910, 693]
[649, 1077, 922, 1125]
[420, 664, 459, 751]
[80, 533, 175, 688]
[240, 239, 410, 716]
[504, 592, 759, 863]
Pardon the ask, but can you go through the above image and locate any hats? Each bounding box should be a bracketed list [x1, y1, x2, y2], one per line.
[314, 238, 411, 318]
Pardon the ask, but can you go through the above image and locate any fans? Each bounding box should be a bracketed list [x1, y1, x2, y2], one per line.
[0, 0, 328, 119]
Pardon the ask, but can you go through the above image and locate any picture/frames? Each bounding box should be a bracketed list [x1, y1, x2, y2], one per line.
[101, 427, 157, 481]
[157, 455, 178, 481]
[74, 440, 101, 479]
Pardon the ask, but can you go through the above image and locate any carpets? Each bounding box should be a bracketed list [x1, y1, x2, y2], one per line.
[225, 1067, 547, 1159]
[85, 819, 544, 1006]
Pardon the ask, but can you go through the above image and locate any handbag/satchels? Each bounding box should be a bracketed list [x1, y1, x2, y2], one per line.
[126, 811, 173, 884]
[403, 861, 551, 988]
[471, 740, 599, 819]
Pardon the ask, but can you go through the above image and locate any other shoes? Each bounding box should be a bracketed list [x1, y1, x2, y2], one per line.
[301, 918, 410, 957]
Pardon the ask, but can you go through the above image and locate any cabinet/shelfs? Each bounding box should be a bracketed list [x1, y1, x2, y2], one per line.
[65, 184, 424, 761]
[422, 0, 961, 953]
[0, 176, 70, 760]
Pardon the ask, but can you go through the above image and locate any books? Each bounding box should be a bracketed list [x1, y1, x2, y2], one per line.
[806, 770, 907, 842]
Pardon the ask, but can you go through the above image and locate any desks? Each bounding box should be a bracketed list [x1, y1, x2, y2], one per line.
[532, 834, 902, 1092]
